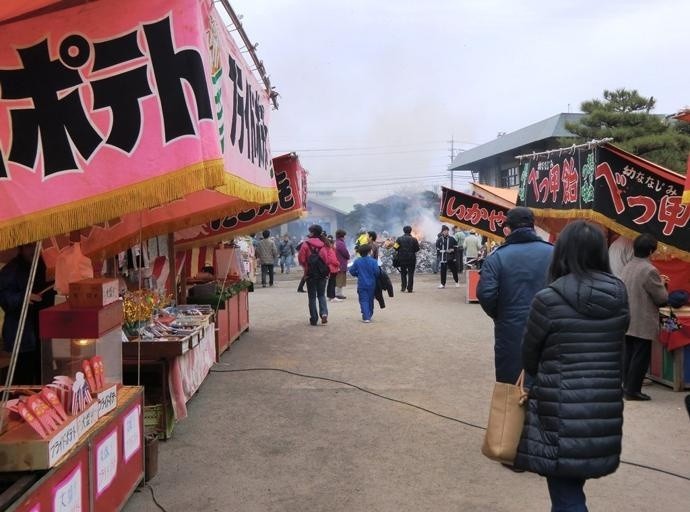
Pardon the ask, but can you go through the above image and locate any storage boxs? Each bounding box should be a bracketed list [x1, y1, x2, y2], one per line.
[69, 275, 120, 308]
[146, 439, 159, 483]
[143, 396, 175, 440]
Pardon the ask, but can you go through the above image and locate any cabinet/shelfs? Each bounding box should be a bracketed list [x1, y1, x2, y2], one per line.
[40, 300, 124, 385]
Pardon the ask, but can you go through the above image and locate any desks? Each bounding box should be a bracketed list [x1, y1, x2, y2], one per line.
[468, 269, 479, 302]
[127, 321, 219, 443]
[0, 385, 146, 512]
[649, 324, 690, 390]
[185, 280, 257, 361]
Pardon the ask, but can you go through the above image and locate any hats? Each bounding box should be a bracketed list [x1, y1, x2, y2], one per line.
[442, 225, 449, 232]
[502, 207, 534, 228]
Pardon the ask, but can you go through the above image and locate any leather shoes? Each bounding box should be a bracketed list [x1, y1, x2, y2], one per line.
[626, 392, 650, 400]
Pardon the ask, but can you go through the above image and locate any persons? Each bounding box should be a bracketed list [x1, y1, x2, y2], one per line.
[607, 234, 635, 381]
[620, 233, 670, 400]
[0, 243, 59, 385]
[254, 225, 482, 326]
[514, 220, 630, 511]
[477, 208, 557, 473]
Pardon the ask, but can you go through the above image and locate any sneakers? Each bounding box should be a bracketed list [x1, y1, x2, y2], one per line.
[455, 282, 459, 287]
[437, 284, 445, 289]
[321, 314, 328, 324]
[310, 321, 317, 325]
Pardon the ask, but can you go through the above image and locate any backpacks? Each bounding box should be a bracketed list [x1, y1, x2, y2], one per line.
[305, 243, 330, 282]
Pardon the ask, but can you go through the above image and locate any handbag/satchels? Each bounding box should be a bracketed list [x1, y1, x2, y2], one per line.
[659, 298, 681, 333]
[482, 369, 530, 467]
[392, 251, 400, 267]
